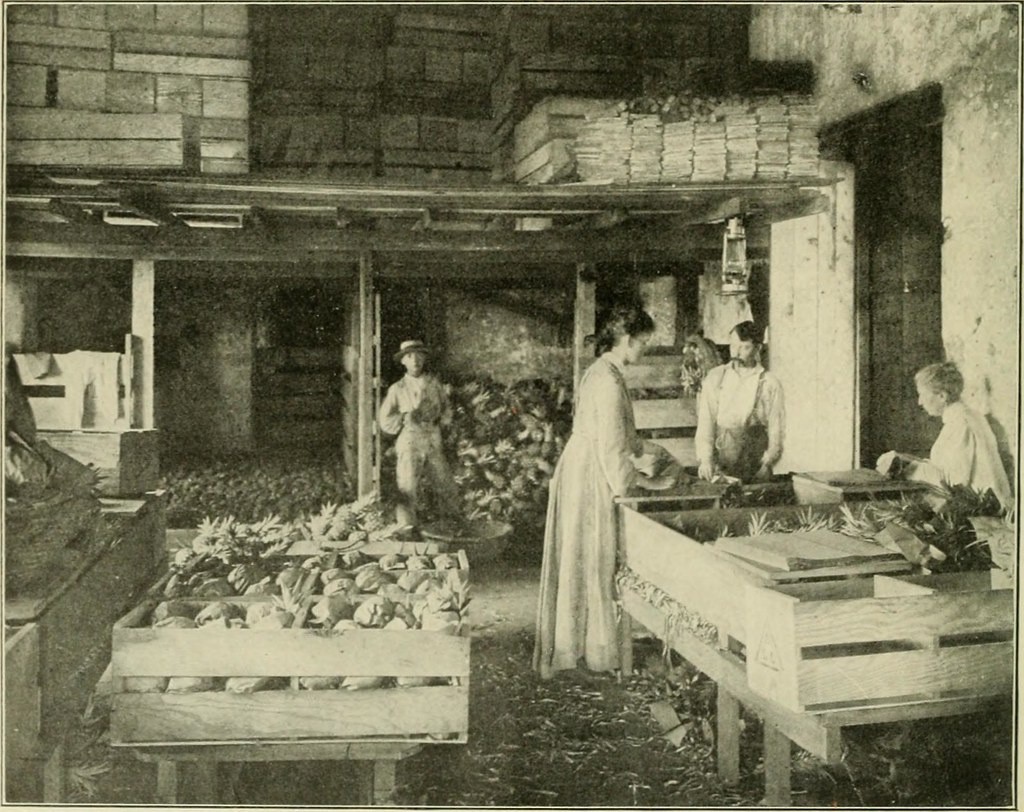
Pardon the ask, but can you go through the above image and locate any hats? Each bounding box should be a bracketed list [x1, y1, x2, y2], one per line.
[392, 337, 432, 362]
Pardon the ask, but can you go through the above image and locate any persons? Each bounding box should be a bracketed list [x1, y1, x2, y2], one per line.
[695, 323, 784, 479]
[876, 362, 1013, 509]
[378, 338, 465, 531]
[529, 307, 657, 677]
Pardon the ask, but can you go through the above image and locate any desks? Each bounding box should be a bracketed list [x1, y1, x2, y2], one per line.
[134, 735, 426, 805]
[614, 584, 1016, 805]
